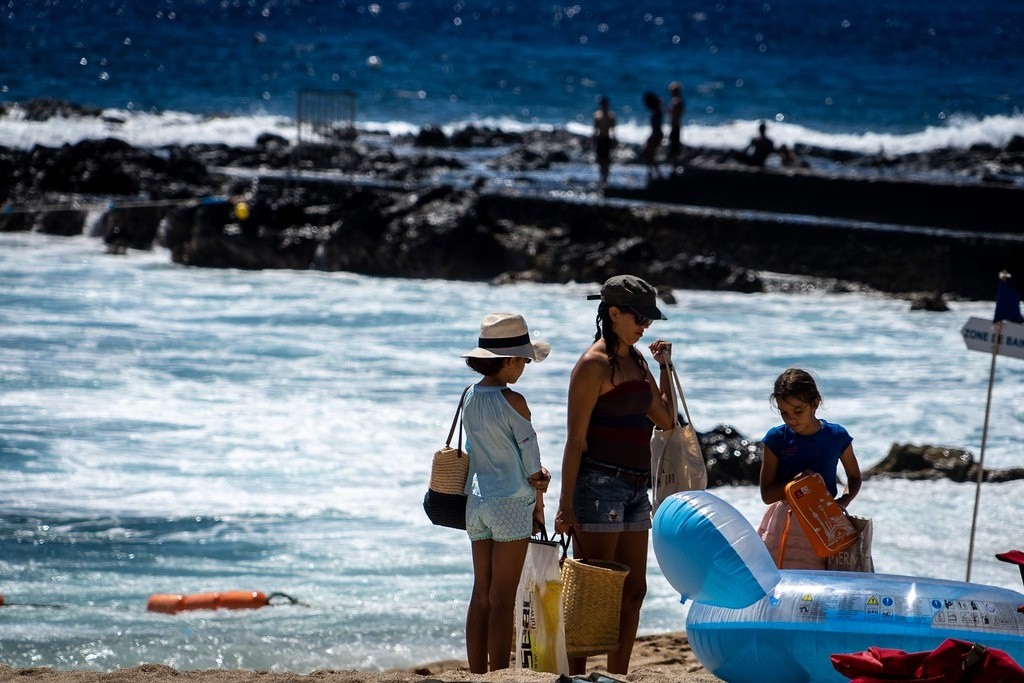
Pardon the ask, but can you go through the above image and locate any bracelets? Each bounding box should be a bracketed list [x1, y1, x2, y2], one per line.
[660, 363, 672, 370]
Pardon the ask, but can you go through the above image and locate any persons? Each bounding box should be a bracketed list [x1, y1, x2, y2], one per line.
[643, 93, 664, 161]
[745, 119, 812, 170]
[593, 97, 617, 189]
[553, 274, 674, 678]
[668, 81, 687, 155]
[459, 314, 547, 670]
[760, 368, 862, 569]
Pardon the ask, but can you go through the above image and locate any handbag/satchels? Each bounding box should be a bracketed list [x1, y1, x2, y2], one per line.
[777, 472, 874, 573]
[651, 350, 707, 518]
[830, 638, 1024, 683]
[516, 539, 569, 677]
[424, 384, 475, 530]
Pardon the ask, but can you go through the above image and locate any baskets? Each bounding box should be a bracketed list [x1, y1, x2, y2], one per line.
[551, 527, 629, 657]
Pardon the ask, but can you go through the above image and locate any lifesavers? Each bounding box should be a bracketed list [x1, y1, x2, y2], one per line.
[143, 586, 269, 614]
[652, 488, 1020, 683]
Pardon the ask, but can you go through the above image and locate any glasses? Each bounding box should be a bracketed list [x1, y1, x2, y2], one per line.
[617, 305, 653, 325]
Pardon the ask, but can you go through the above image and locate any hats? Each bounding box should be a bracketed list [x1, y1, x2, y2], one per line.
[461, 313, 551, 362]
[587, 275, 667, 322]
